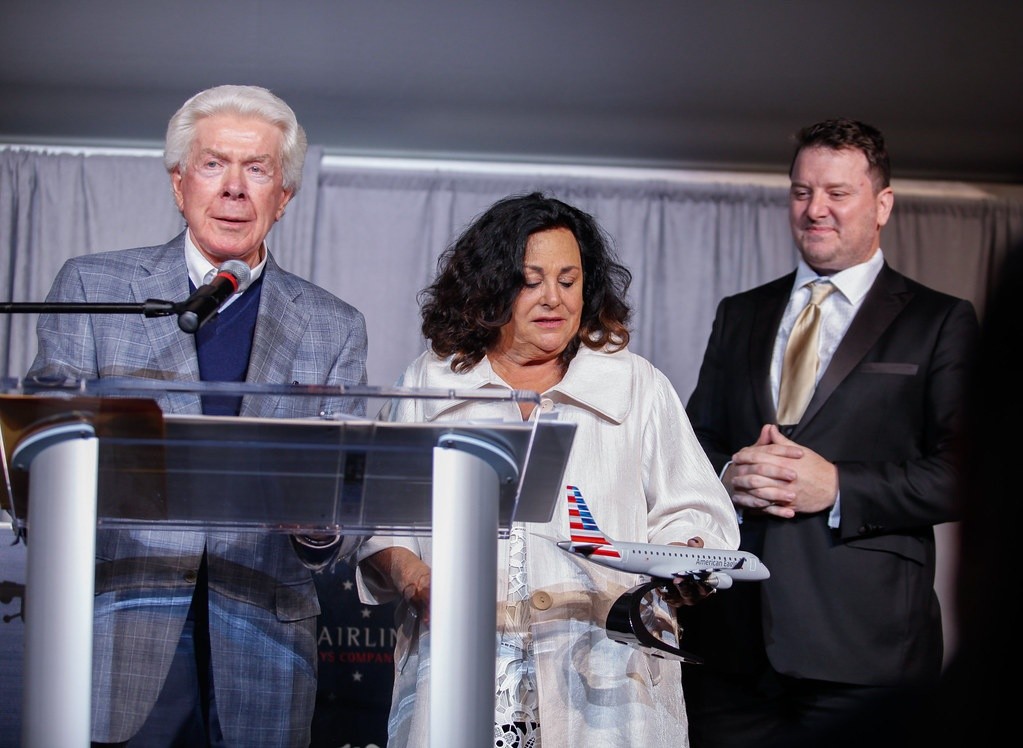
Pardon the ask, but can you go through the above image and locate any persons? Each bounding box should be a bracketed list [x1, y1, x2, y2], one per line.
[682, 116, 981, 748]
[22, 83, 367, 748]
[351, 191, 741, 748]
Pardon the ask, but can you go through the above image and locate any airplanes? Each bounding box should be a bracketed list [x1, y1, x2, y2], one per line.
[555, 484, 772, 589]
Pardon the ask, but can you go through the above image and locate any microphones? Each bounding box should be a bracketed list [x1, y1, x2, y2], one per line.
[178, 259, 251, 334]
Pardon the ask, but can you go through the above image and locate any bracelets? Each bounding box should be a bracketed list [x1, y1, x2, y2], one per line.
[302, 536, 335, 545]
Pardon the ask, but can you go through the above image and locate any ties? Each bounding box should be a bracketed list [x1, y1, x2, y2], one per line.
[776, 282, 840, 425]
